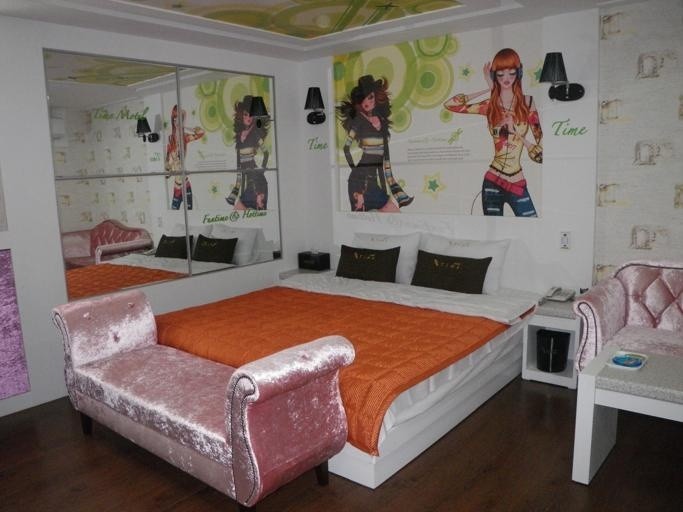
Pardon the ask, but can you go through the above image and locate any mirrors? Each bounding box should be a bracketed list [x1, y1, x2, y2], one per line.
[41, 47, 283, 305]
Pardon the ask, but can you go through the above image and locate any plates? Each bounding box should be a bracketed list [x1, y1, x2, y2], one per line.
[607, 350, 646, 370]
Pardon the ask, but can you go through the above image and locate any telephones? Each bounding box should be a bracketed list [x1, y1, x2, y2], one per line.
[544, 286, 575, 302]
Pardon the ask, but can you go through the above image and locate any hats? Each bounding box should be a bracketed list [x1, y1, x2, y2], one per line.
[237, 95, 254, 113]
[349, 74, 384, 102]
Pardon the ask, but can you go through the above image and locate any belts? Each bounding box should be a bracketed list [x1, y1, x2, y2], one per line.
[483, 170, 527, 196]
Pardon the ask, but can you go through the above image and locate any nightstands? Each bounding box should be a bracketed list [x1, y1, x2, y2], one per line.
[279, 268, 332, 280]
[521, 301, 583, 390]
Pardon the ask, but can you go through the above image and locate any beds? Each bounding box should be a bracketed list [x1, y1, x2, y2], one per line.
[154, 271, 541, 490]
[52, 291, 355, 512]
[66, 249, 235, 301]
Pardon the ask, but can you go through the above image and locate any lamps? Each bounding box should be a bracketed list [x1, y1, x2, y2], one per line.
[539, 52, 585, 101]
[249, 97, 270, 129]
[136, 117, 159, 142]
[305, 87, 326, 124]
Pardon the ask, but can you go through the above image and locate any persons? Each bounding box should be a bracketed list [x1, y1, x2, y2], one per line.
[162, 104, 205, 212]
[444, 47, 545, 217]
[332, 73, 416, 214]
[223, 94, 271, 215]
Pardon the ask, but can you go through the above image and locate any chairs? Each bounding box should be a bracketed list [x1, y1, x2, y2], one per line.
[574, 260, 683, 374]
[61, 220, 153, 269]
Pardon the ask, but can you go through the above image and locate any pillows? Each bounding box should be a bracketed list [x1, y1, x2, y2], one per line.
[354, 232, 421, 285]
[410, 249, 493, 294]
[211, 224, 258, 266]
[155, 234, 193, 259]
[421, 232, 512, 295]
[193, 234, 238, 263]
[336, 245, 400, 283]
[171, 223, 212, 239]
